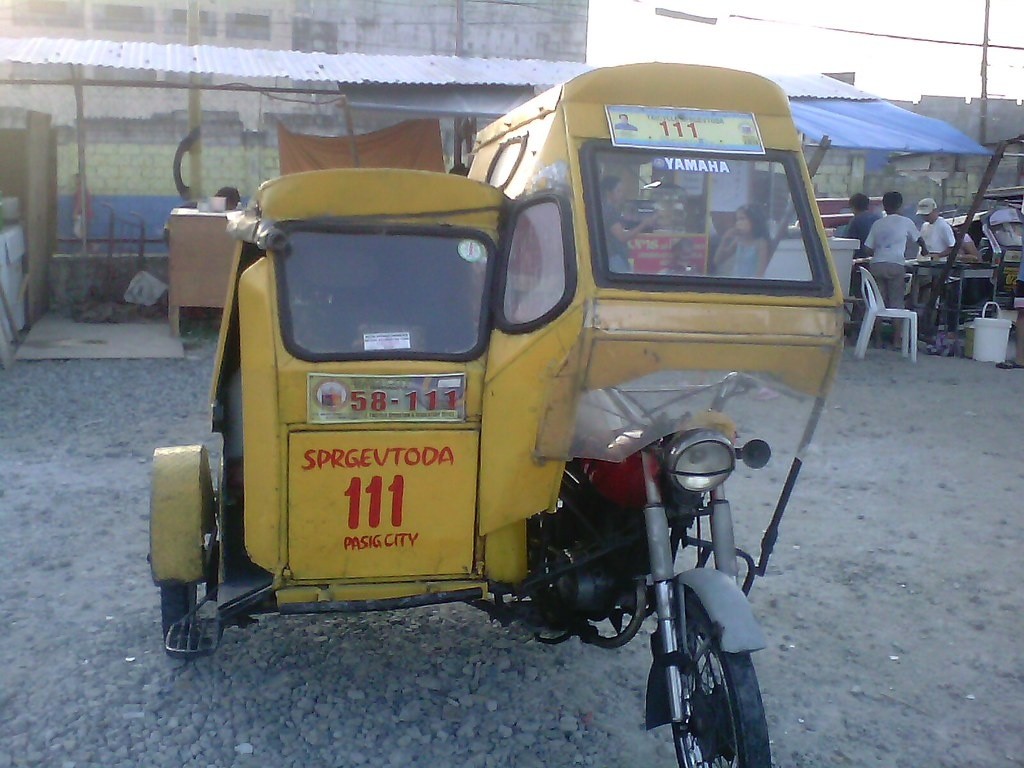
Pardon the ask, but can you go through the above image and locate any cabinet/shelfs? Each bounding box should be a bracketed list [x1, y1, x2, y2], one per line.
[765, 238, 863, 335]
[168, 208, 237, 336]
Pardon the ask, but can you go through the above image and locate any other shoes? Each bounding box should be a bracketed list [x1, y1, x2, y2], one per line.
[927, 346, 938, 354]
[996, 361, 1024, 370]
[873, 345, 891, 351]
[892, 345, 919, 353]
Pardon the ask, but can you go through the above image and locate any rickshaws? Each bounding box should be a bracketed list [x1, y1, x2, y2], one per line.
[147, 62, 843, 767]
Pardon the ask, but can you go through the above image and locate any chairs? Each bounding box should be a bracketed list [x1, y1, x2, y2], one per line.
[854, 266, 917, 361]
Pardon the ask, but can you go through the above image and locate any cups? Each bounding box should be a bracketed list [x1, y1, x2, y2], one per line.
[197, 202, 209, 212]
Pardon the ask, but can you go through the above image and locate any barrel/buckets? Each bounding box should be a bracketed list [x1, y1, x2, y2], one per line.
[972, 301, 1012, 363]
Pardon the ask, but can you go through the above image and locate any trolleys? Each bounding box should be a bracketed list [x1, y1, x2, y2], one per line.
[100, 202, 169, 321]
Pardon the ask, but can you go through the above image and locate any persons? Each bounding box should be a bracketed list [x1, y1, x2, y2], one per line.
[616, 114, 637, 131]
[915, 198, 981, 335]
[599, 175, 771, 279]
[843, 193, 880, 259]
[996, 199, 1024, 369]
[864, 192, 928, 351]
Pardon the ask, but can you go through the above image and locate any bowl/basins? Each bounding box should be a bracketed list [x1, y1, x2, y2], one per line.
[208, 197, 226, 212]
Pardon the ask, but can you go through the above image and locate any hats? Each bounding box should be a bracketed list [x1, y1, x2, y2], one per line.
[216, 187, 241, 203]
[916, 198, 937, 214]
[844, 194, 868, 207]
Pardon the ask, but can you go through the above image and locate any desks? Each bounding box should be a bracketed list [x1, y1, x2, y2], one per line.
[854, 261, 995, 359]
